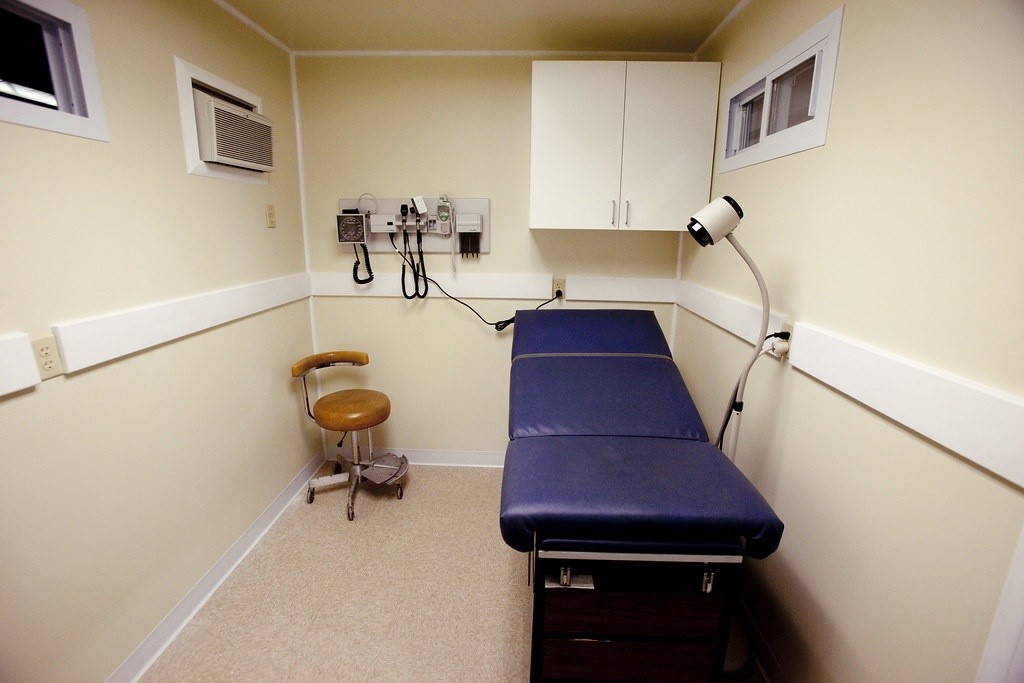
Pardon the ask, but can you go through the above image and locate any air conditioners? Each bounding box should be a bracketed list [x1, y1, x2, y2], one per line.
[193, 88, 275, 174]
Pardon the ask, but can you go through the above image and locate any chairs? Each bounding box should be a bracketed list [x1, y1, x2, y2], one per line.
[290, 350, 409, 516]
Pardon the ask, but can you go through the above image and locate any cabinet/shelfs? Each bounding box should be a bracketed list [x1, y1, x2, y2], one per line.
[531, 59, 722, 234]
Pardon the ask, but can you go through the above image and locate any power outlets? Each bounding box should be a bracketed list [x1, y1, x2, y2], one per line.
[777, 321, 793, 361]
[33, 334, 63, 384]
[267, 204, 276, 229]
[553, 278, 565, 300]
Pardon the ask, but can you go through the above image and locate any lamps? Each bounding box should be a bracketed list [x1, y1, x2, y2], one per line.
[687, 194, 770, 463]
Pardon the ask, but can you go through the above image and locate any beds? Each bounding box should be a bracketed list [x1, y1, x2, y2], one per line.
[499, 311, 787, 683]
